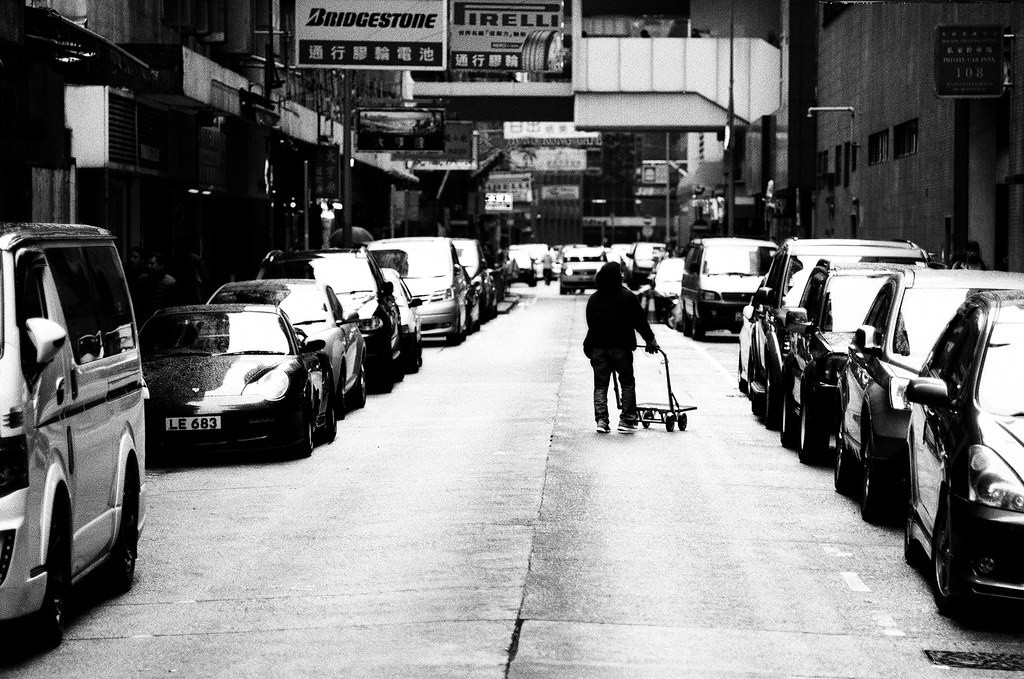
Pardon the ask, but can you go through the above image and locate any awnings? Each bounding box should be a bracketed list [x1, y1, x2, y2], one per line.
[676, 162, 726, 200]
[25, 6, 147, 81]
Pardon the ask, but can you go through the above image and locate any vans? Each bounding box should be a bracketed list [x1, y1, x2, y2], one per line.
[679, 237, 780, 342]
[0, 219, 149, 648]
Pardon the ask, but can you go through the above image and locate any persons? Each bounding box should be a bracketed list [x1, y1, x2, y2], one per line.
[583, 261, 660, 434]
[542, 254, 553, 285]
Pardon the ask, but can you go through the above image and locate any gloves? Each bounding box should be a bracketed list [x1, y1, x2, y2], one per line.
[645, 338, 660, 354]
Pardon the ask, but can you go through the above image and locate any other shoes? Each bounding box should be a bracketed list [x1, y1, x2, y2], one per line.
[596, 419, 611, 432]
[617, 420, 638, 432]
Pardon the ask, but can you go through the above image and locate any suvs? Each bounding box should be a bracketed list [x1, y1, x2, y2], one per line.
[254, 246, 403, 395]
[735, 236, 934, 431]
[368, 236, 474, 345]
[450, 239, 500, 334]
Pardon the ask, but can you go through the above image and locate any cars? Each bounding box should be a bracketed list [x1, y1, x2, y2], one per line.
[772, 262, 938, 468]
[904, 288, 1024, 621]
[834, 266, 1024, 526]
[138, 302, 327, 469]
[194, 278, 368, 421]
[381, 267, 424, 374]
[507, 239, 693, 330]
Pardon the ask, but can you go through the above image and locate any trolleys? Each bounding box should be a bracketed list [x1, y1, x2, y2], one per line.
[612, 344, 698, 432]
